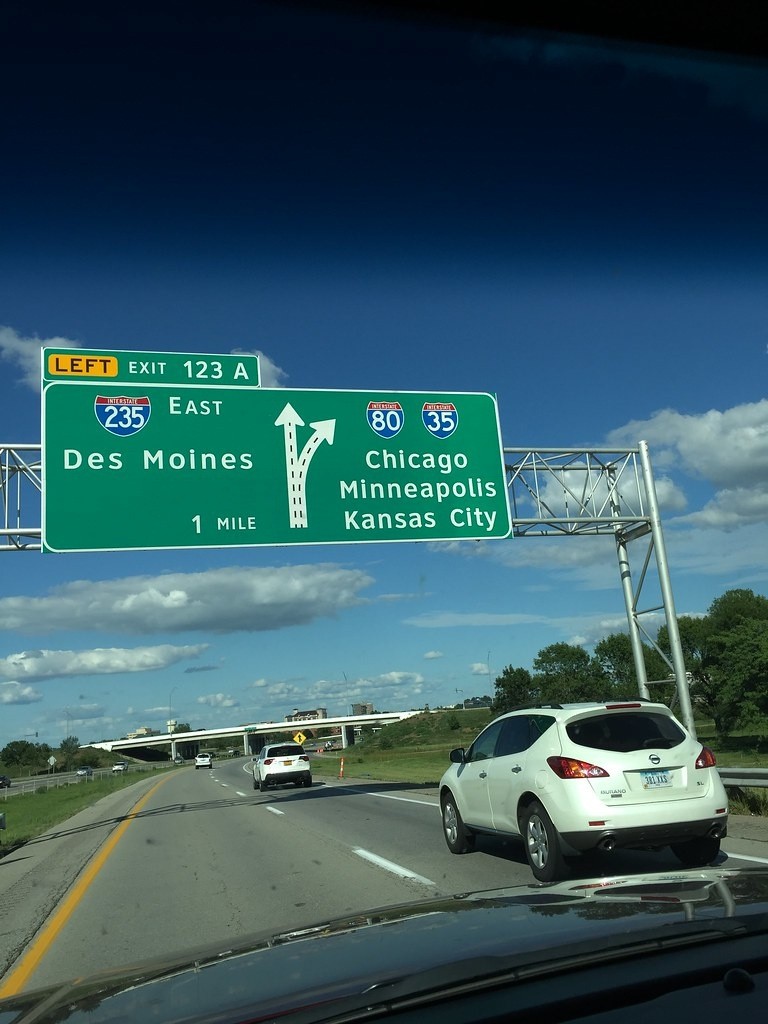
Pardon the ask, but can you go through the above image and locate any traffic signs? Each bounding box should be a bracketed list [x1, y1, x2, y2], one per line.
[38, 347, 515, 556]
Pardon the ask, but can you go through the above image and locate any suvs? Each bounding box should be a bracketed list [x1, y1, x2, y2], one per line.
[438, 700, 730, 883]
[251, 742, 313, 792]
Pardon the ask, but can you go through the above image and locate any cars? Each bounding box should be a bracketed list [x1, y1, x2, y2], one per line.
[194, 752, 213, 770]
[76, 765, 93, 776]
[0, 775, 11, 789]
[112, 761, 128, 773]
[174, 755, 184, 764]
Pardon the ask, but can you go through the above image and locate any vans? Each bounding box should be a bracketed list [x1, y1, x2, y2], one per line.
[229, 750, 241, 757]
